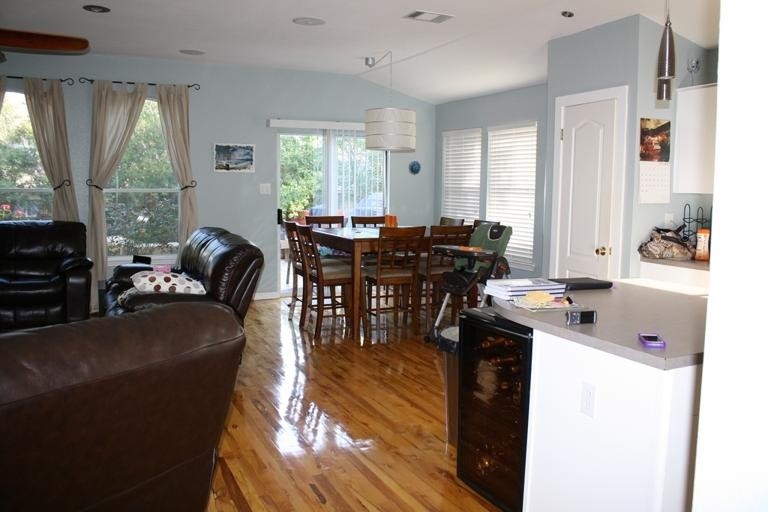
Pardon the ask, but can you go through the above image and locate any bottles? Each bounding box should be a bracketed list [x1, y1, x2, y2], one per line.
[695, 229, 710, 261]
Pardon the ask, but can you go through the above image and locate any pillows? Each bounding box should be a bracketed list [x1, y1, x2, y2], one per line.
[117, 271, 206, 308]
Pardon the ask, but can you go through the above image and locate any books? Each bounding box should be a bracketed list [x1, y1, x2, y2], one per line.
[485, 276, 614, 312]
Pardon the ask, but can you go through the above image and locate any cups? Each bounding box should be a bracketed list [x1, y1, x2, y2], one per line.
[385, 215, 397, 228]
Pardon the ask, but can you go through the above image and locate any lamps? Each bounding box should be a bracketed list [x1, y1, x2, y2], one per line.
[657, 1, 675, 99]
[365, 50, 416, 152]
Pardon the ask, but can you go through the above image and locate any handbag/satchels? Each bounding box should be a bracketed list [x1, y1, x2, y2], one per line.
[638, 224, 696, 261]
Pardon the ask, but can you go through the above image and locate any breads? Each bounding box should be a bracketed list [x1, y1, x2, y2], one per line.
[527, 290, 553, 303]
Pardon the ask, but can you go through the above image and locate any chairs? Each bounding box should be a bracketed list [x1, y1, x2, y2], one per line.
[283, 216, 512, 341]
[1, 222, 93, 332]
[0, 300, 246, 512]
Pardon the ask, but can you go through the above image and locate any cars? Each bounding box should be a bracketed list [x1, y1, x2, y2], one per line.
[356, 188, 384, 219]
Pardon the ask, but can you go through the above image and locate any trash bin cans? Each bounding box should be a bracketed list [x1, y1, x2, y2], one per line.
[438, 325, 461, 448]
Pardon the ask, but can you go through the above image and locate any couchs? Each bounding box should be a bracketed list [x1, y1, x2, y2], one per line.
[101, 227, 263, 320]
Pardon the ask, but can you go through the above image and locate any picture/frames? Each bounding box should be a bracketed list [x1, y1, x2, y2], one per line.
[214, 143, 255, 173]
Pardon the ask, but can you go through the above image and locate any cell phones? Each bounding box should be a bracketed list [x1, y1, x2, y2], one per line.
[565, 310, 597, 325]
[638, 333, 666, 349]
